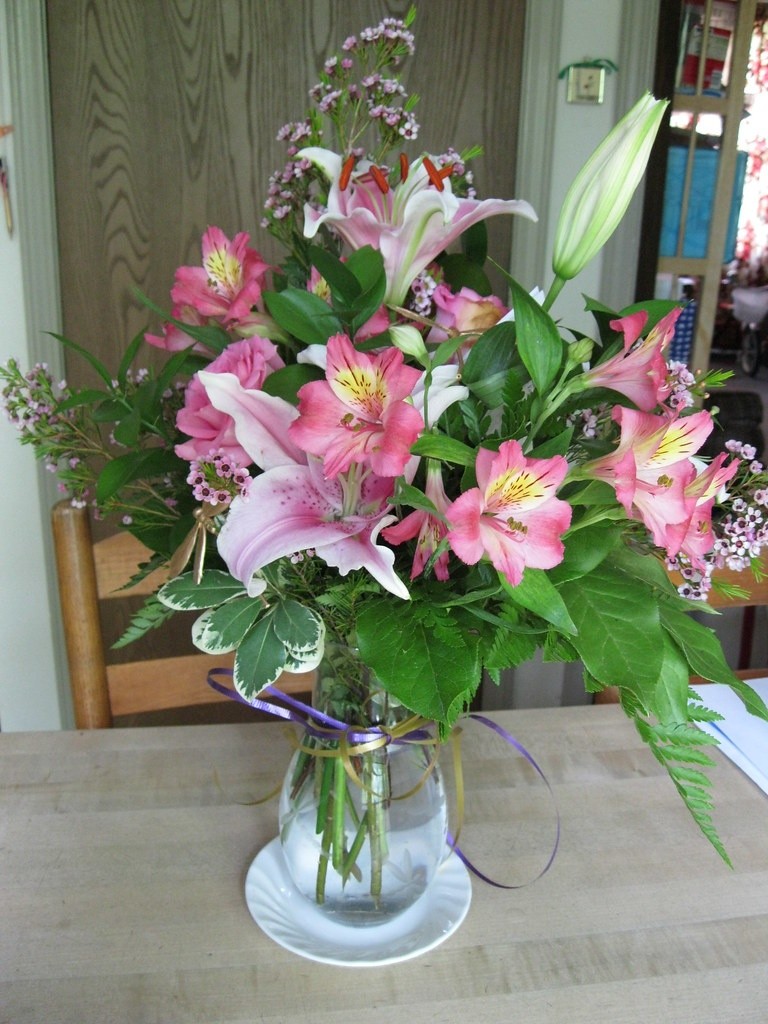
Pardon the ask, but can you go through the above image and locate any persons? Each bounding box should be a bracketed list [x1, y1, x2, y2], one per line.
[751, 265, 768, 287]
[727, 258, 748, 289]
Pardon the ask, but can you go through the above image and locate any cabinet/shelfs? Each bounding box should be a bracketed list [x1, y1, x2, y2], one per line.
[639, 1, 756, 409]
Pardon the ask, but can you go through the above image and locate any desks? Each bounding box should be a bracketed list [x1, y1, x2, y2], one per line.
[1, 700, 765, 1022]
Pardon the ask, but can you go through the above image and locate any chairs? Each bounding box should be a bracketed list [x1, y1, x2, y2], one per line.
[34, 486, 316, 728]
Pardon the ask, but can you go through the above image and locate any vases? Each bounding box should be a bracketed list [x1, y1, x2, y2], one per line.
[275, 634, 447, 926]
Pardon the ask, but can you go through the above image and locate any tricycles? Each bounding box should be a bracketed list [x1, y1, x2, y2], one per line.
[682, 265, 768, 374]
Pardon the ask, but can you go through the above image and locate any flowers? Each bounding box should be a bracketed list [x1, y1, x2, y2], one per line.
[0, 1, 767, 919]
